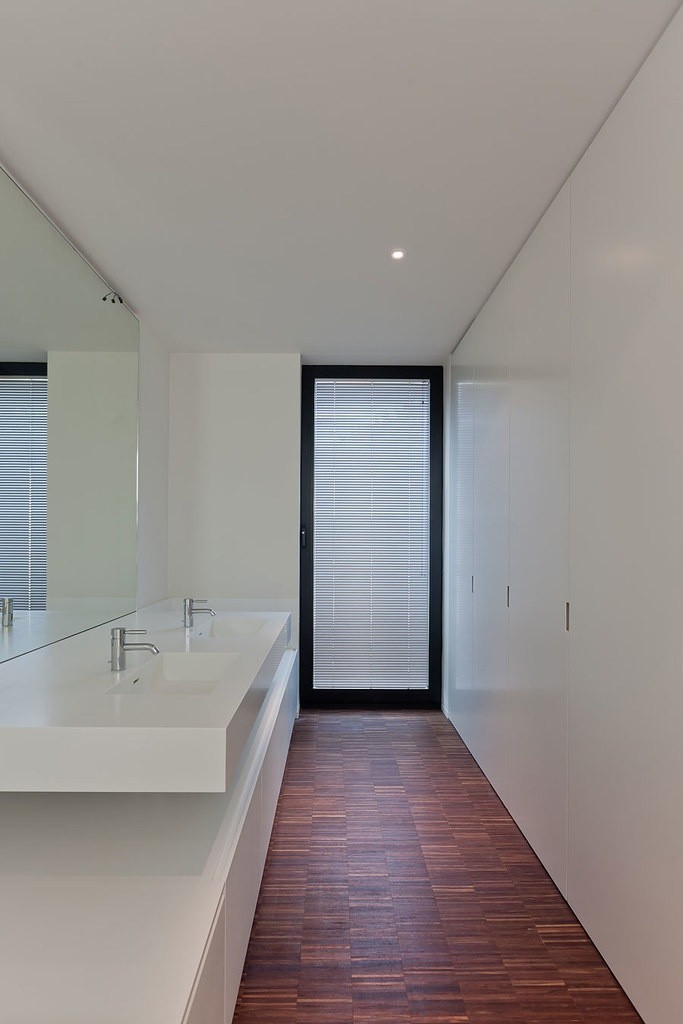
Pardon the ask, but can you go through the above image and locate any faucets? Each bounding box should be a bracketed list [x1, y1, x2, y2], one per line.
[109, 627, 161, 671]
[0, 596, 15, 626]
[181, 598, 217, 627]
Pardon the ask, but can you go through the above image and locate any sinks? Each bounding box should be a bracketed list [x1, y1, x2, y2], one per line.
[0, 616, 23, 628]
[187, 614, 268, 638]
[102, 652, 247, 696]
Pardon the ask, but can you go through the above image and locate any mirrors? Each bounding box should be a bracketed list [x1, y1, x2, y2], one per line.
[0, 162, 139, 662]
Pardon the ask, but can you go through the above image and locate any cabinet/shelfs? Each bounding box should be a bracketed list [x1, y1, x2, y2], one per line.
[0, 645, 302, 1024]
